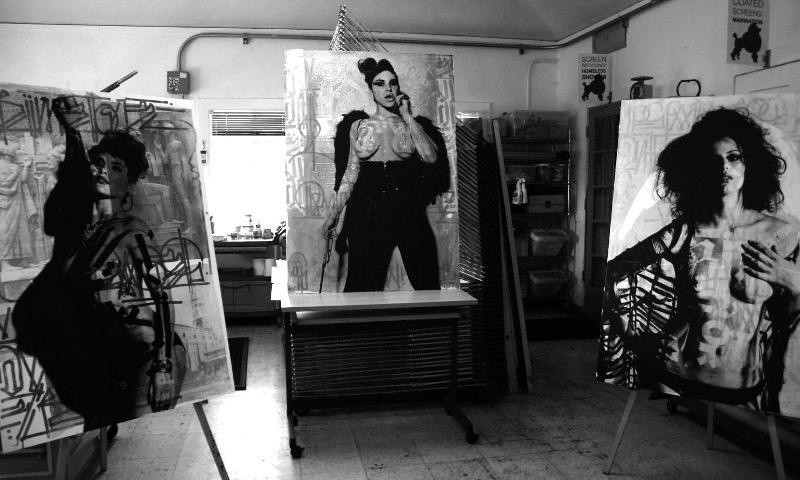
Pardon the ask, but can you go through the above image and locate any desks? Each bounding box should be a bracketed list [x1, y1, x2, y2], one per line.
[277, 288, 483, 456]
[209, 230, 284, 330]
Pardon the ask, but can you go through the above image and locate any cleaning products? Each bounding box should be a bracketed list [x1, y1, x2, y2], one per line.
[513, 177, 528, 204]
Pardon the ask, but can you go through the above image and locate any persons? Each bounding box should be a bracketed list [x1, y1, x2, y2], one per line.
[601, 107, 799, 399]
[1, 116, 202, 261]
[312, 56, 450, 291]
[62, 127, 187, 415]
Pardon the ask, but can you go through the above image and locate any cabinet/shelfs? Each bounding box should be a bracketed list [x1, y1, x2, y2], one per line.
[583, 102, 636, 321]
[496, 110, 577, 324]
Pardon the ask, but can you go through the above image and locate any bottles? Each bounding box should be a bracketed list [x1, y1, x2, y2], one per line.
[512, 177, 529, 206]
[230, 213, 272, 240]
